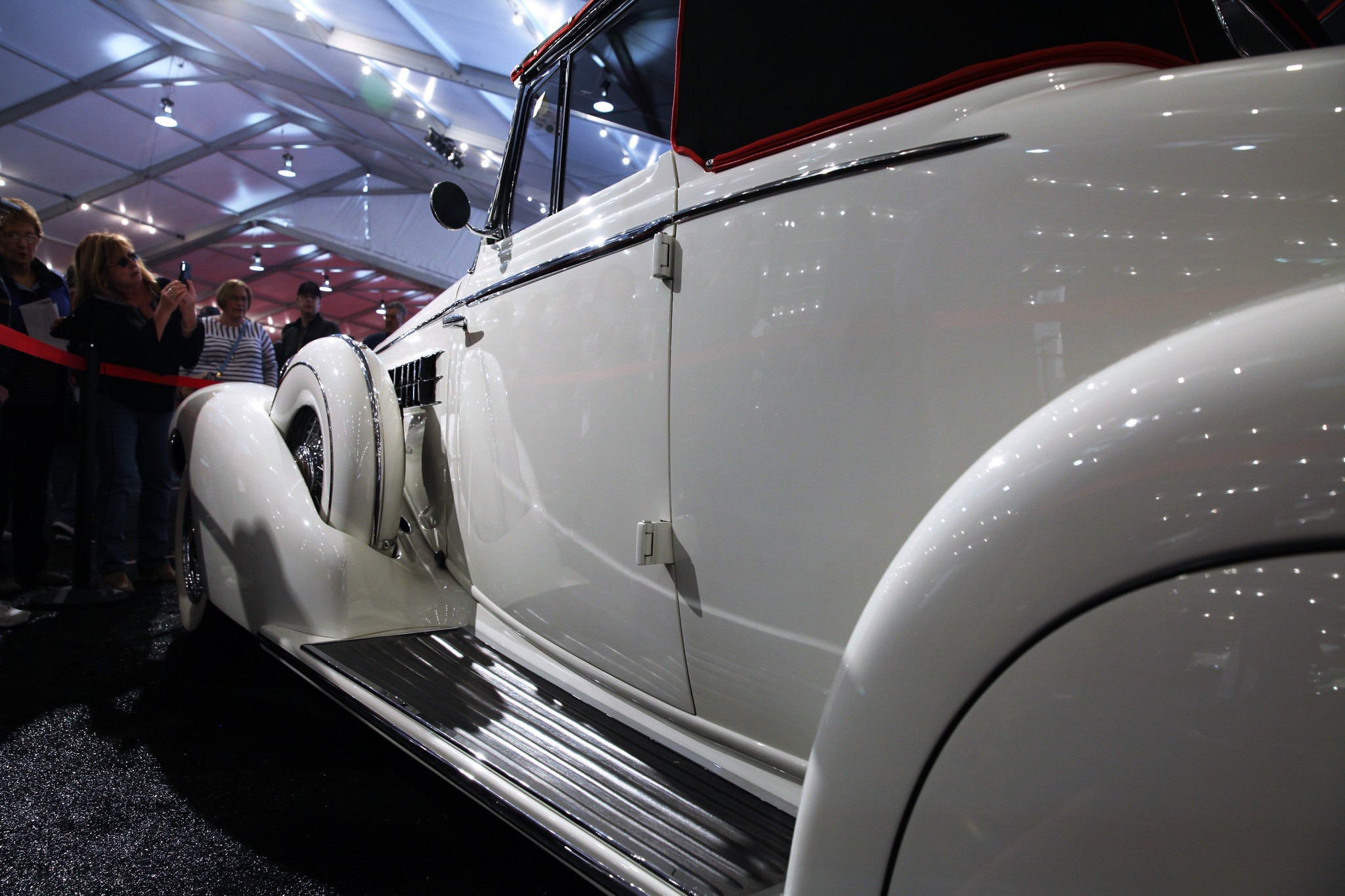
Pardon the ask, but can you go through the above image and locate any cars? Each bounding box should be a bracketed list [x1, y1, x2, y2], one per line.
[135, 1, 1345, 895]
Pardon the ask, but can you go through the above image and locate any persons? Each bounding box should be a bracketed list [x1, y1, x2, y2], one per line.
[49, 227, 205, 592]
[362, 302, 407, 350]
[273, 322, 294, 374]
[179, 282, 279, 400]
[51, 263, 76, 542]
[280, 282, 338, 373]
[0, 196, 81, 626]
[197, 307, 221, 318]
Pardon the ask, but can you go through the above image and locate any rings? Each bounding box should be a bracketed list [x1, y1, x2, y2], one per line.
[174, 296, 178, 301]
[167, 288, 173, 295]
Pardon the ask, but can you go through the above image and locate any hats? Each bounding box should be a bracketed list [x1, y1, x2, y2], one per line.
[298, 282, 322, 298]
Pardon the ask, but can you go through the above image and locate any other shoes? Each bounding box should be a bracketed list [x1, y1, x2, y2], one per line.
[36, 570, 68, 586]
[2, 531, 13, 542]
[0, 573, 21, 592]
[136, 562, 176, 583]
[52, 520, 75, 540]
[0, 601, 31, 626]
[99, 575, 139, 596]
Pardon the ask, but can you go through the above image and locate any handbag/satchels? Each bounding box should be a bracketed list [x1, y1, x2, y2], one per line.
[176, 371, 221, 404]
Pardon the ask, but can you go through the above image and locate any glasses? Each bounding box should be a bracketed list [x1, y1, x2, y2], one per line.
[0, 231, 36, 241]
[109, 253, 137, 270]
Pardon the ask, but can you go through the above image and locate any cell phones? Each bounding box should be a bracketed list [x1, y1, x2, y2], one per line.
[180, 262, 190, 290]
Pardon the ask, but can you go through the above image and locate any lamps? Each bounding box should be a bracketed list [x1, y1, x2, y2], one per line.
[424, 131, 464, 169]
[249, 253, 264, 271]
[276, 152, 297, 178]
[318, 273, 332, 291]
[591, 78, 616, 114]
[376, 299, 386, 315]
[155, 96, 178, 127]
